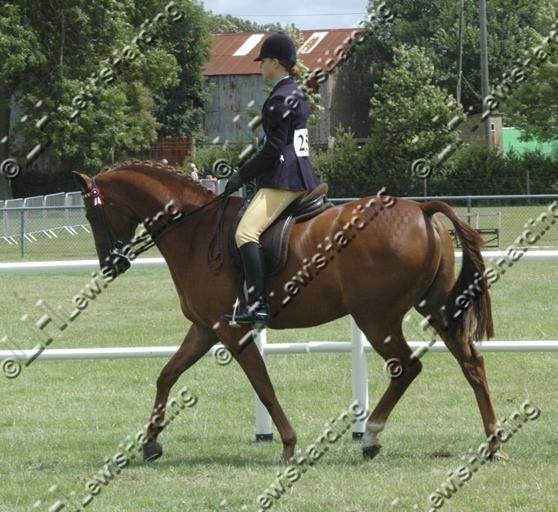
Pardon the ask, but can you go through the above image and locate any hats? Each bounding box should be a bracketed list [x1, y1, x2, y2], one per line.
[254, 32, 296, 62]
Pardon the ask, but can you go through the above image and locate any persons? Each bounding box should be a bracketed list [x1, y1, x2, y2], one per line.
[221, 31, 320, 324]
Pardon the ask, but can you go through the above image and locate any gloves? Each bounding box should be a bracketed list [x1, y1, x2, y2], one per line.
[225, 172, 245, 193]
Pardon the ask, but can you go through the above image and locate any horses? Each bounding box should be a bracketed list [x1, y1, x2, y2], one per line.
[70, 159, 512, 464]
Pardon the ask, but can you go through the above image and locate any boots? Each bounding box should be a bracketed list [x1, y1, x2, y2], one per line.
[221, 241, 270, 324]
